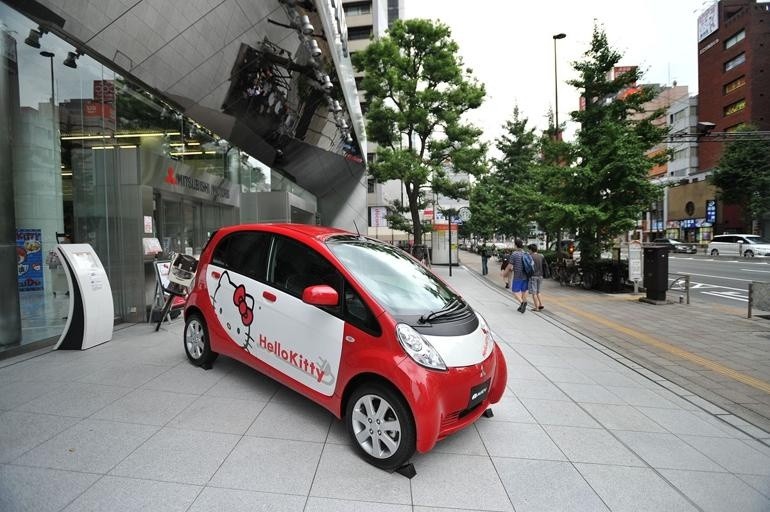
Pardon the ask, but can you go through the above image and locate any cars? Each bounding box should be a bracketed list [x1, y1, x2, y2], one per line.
[651, 233, 770, 258]
[184, 220, 508, 472]
[550, 239, 581, 260]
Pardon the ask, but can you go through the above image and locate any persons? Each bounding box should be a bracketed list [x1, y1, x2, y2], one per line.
[482, 244, 491, 275]
[396, 241, 410, 253]
[501, 240, 550, 313]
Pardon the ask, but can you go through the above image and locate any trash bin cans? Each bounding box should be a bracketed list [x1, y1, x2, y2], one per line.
[643, 246, 670, 301]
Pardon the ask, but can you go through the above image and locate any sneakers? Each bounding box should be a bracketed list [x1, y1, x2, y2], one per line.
[517, 302, 527, 313]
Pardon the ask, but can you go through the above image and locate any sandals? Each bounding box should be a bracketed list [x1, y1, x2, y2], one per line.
[532, 305, 544, 311]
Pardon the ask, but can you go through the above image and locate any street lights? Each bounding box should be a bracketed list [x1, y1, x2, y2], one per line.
[553, 33, 566, 256]
[39, 50, 55, 111]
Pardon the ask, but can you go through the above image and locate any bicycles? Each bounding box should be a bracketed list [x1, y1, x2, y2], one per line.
[551, 257, 600, 290]
[155, 253, 199, 332]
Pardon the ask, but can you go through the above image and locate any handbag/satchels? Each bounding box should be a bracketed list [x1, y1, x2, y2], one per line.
[522, 253, 535, 276]
[543, 264, 550, 279]
